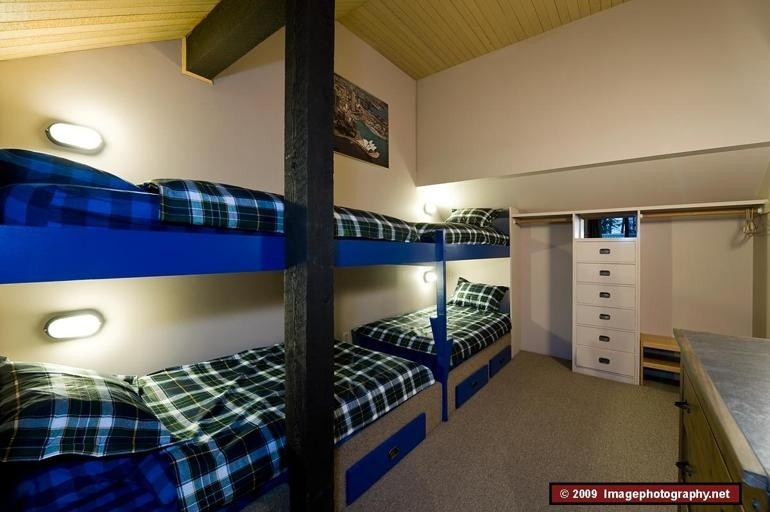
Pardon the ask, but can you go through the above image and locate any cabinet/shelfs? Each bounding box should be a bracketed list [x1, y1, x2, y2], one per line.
[572, 210, 641, 386]
[673, 328, 770, 512]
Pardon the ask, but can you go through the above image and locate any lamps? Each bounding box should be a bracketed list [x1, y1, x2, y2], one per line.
[424, 202, 438, 217]
[44, 122, 105, 156]
[424, 270, 438, 283]
[42, 309, 104, 341]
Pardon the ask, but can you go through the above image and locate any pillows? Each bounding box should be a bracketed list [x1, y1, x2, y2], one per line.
[446, 277, 509, 314]
[0, 356, 190, 462]
[0, 148, 144, 193]
[444, 208, 502, 229]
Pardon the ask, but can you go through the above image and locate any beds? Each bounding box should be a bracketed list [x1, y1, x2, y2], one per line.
[0, 177, 443, 512]
[351, 220, 511, 423]
